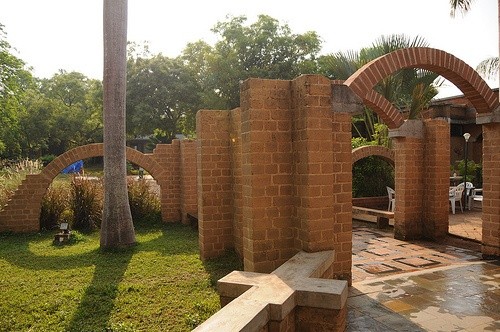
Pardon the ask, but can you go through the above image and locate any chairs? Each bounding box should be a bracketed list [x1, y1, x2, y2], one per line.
[455, 181, 475, 208]
[385, 185, 396, 212]
[448, 187, 465, 215]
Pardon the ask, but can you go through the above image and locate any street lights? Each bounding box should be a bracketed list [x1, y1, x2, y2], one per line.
[463, 131, 472, 212]
